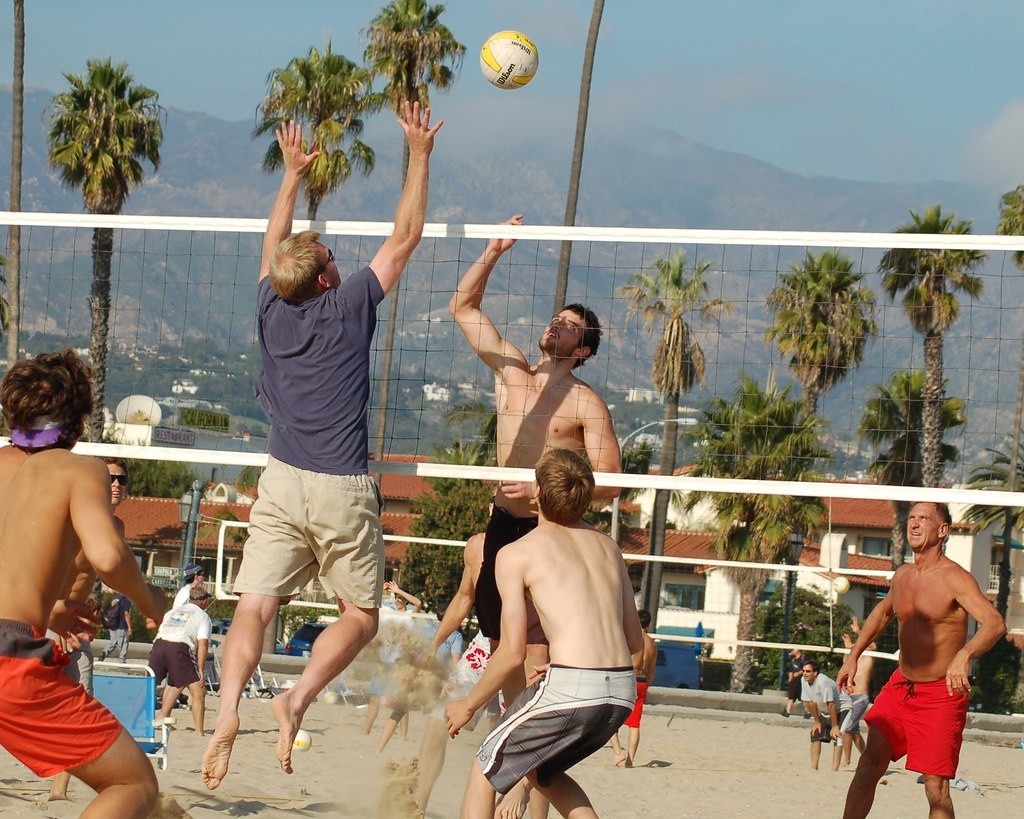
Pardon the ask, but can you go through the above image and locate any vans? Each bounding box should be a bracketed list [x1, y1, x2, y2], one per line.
[645, 640, 700, 691]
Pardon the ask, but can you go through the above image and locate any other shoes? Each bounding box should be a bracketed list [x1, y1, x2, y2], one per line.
[98, 651, 105, 661]
[780, 712, 789, 717]
[804, 712, 811, 719]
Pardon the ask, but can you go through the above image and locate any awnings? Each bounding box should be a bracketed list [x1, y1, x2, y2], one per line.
[991, 534, 1024, 552]
[760, 580, 782, 595]
[655, 624, 714, 639]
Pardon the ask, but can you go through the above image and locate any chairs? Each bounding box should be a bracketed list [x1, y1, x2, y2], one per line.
[92, 661, 177, 770]
[326, 669, 368, 707]
[204, 652, 286, 698]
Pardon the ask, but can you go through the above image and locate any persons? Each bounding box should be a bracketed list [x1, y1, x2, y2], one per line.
[0, 350, 165, 819]
[45, 456, 127, 803]
[450, 215, 623, 819]
[199, 100, 444, 789]
[445, 448, 646, 819]
[836, 500, 1007, 819]
[97, 564, 213, 741]
[780, 616, 876, 772]
[362, 487, 507, 819]
[610, 609, 657, 767]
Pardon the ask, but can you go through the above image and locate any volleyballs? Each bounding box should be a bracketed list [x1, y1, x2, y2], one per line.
[478, 29, 541, 89]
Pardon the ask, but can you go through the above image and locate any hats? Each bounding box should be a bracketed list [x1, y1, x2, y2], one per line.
[190, 592, 212, 600]
[179, 565, 203, 581]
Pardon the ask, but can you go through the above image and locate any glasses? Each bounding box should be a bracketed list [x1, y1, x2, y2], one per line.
[803, 670, 811, 673]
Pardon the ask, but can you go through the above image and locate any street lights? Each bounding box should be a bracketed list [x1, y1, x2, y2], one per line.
[610, 419, 699, 541]
[778, 526, 805, 690]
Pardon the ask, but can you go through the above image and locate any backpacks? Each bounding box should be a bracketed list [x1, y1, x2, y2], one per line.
[101, 598, 125, 630]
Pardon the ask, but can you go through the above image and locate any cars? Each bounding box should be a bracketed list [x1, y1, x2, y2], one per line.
[285, 620, 332, 659]
[275, 638, 286, 655]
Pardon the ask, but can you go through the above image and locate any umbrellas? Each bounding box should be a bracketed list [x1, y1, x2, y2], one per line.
[691, 621, 704, 658]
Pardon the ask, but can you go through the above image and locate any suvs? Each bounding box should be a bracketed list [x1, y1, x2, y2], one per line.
[210, 616, 232, 635]
